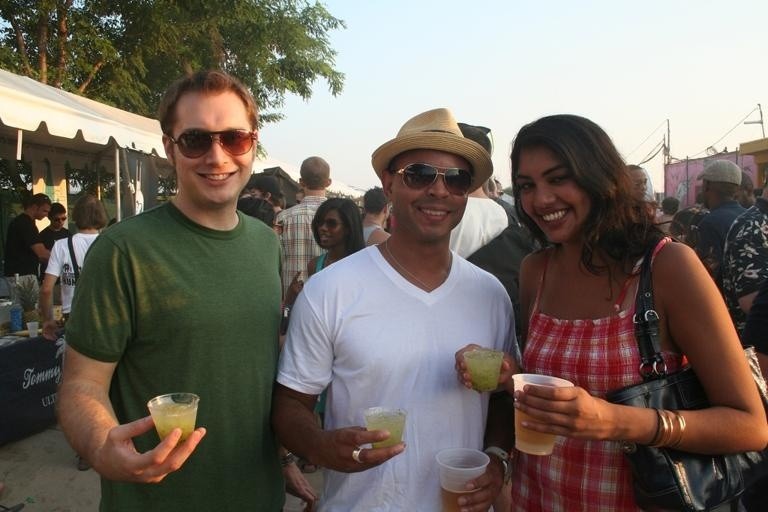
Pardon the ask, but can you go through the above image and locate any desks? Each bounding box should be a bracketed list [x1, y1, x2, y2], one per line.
[0, 324, 64, 448]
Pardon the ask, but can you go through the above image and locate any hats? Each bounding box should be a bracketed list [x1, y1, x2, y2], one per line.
[373, 108, 494, 194]
[697, 159, 742, 186]
[248, 174, 285, 195]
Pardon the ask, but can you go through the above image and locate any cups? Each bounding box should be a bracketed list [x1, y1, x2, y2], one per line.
[435, 449, 490, 512]
[512, 373, 574, 455]
[361, 406, 408, 447]
[463, 350, 504, 391]
[147, 393, 201, 443]
[26, 322, 38, 338]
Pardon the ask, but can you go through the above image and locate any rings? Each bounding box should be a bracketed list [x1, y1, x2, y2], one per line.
[350, 448, 365, 463]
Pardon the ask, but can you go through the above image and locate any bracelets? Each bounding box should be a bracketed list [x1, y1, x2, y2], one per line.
[651, 407, 686, 449]
[484, 446, 512, 486]
[279, 454, 295, 467]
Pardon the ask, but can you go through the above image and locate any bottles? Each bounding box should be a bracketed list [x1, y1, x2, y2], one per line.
[10, 305, 24, 332]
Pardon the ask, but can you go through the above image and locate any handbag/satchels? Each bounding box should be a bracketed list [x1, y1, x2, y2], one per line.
[606, 345, 768, 511]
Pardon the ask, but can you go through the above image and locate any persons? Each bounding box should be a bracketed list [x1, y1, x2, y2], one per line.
[455, 115, 768, 510]
[4, 192, 109, 343]
[57, 72, 318, 512]
[237, 123, 514, 318]
[628, 159, 768, 373]
[271, 108, 522, 511]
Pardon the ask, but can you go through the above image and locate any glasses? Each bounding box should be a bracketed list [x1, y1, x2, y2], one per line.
[54, 216, 67, 223]
[396, 165, 472, 196]
[315, 219, 344, 229]
[169, 129, 256, 158]
